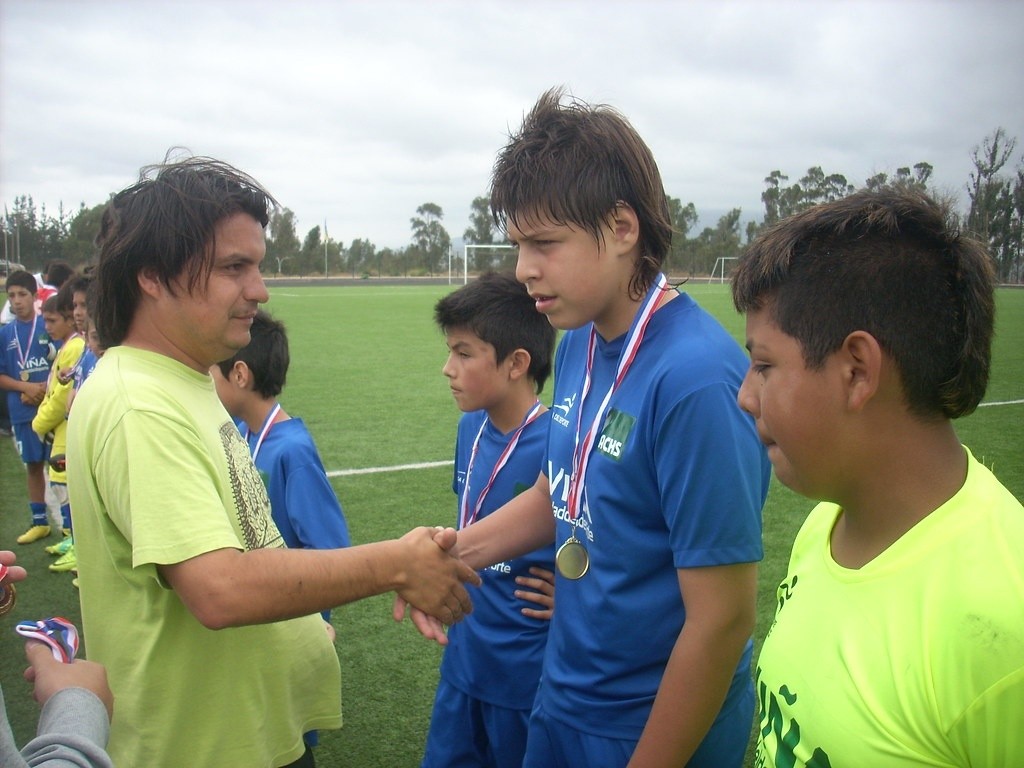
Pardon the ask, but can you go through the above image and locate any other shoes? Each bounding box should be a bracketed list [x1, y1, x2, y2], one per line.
[0, 428, 13, 436]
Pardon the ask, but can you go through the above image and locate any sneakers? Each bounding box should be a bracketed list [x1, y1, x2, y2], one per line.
[48, 546, 79, 572]
[62, 528, 72, 539]
[17, 525, 51, 544]
[71, 567, 79, 589]
[46, 537, 74, 554]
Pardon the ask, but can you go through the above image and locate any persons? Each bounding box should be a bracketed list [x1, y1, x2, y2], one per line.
[420, 269, 556, 768]
[0, 258, 353, 747]
[64, 156, 483, 768]
[0, 549, 117, 768]
[730, 190, 1023, 768]
[392, 87, 772, 768]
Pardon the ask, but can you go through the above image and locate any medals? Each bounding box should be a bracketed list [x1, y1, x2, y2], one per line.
[20, 372, 29, 382]
[556, 536, 588, 580]
[0, 583, 17, 618]
